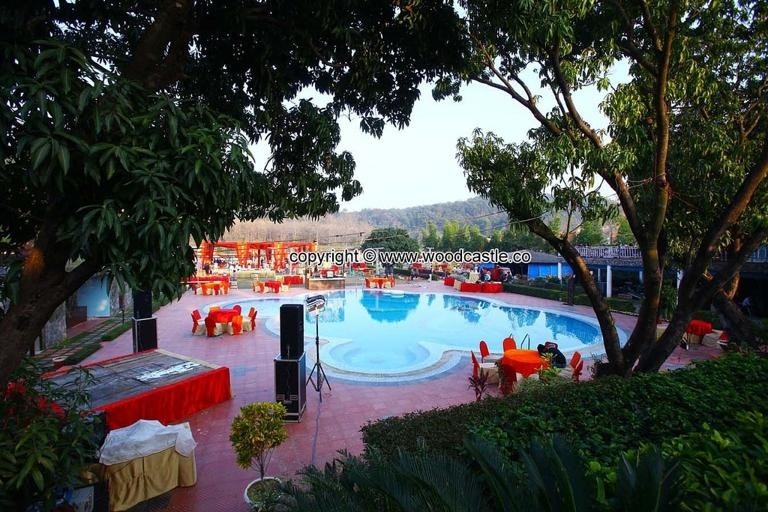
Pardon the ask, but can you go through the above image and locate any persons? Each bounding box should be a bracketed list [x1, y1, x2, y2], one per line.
[231, 270, 237, 281]
[537, 344, 566, 368]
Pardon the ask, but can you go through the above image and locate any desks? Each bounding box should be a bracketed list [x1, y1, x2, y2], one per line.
[686, 320, 712, 345]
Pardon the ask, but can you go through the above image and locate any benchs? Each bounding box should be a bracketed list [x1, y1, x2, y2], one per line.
[707, 349, 723, 359]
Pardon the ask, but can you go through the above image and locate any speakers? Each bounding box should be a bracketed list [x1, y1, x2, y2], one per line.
[133, 287, 152, 319]
[132, 316, 157, 352]
[273, 352, 306, 423]
[280, 304, 304, 360]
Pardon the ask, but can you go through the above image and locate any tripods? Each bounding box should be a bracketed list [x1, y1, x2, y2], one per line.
[306, 315, 332, 402]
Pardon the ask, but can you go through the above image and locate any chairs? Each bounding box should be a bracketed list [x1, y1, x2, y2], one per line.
[190, 304, 257, 336]
[181, 273, 231, 296]
[470, 337, 583, 399]
[244, 260, 396, 294]
[443, 275, 504, 293]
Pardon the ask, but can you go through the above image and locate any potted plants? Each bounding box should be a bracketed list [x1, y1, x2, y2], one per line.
[229, 400, 288, 512]
